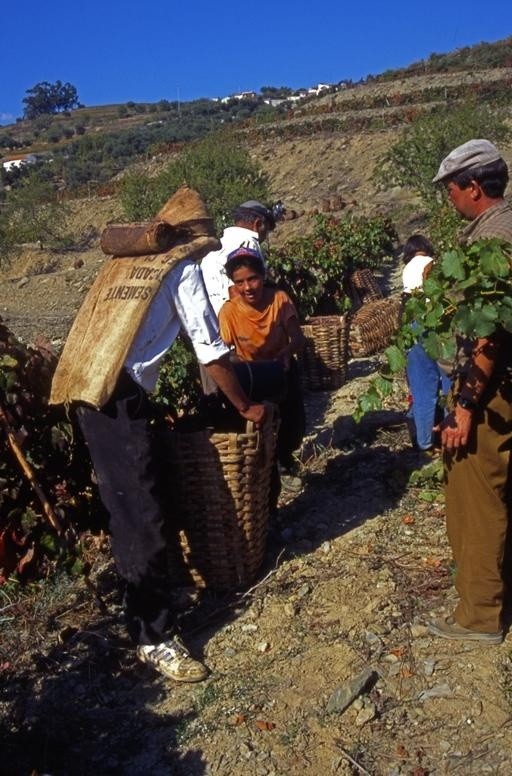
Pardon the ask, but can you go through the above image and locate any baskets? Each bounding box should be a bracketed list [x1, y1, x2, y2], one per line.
[174, 410, 281, 592]
[352, 269, 383, 303]
[300, 315, 349, 390]
[351, 299, 401, 358]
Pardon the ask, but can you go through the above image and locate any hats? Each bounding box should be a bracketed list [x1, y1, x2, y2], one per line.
[432, 138, 502, 183]
[227, 248, 261, 260]
[236, 200, 275, 230]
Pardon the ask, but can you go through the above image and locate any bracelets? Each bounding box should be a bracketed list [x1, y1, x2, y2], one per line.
[456, 396, 478, 412]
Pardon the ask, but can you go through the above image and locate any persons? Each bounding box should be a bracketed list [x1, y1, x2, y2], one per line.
[423, 136, 511, 647]
[398, 234, 455, 458]
[60, 188, 269, 683]
[192, 200, 277, 401]
[214, 246, 308, 471]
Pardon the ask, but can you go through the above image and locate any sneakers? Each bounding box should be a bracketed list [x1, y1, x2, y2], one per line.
[425, 616, 504, 644]
[137, 635, 208, 681]
